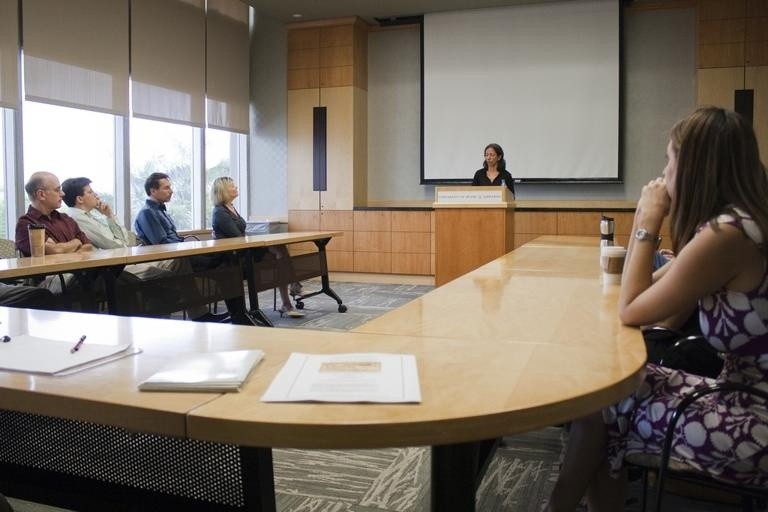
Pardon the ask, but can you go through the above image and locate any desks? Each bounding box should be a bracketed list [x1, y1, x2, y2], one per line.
[0, 233, 649, 512]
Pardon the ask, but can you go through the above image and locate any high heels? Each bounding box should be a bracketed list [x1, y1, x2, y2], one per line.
[278, 304, 306, 318]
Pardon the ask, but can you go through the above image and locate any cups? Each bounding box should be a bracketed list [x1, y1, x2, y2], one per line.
[28, 223, 47, 258]
[601, 246, 626, 284]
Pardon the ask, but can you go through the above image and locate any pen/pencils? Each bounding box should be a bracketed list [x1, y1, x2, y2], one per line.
[70, 335, 86, 353]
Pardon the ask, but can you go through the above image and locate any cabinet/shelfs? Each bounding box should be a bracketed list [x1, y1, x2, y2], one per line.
[283, 16, 370, 211]
[695, 1, 765, 166]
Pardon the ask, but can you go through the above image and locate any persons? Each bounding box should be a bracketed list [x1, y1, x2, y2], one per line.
[208, 174, 306, 320]
[133, 169, 249, 318]
[544, 103, 768, 511]
[0, 281, 54, 309]
[639, 248, 704, 338]
[471, 142, 515, 199]
[12, 170, 146, 318]
[58, 175, 232, 322]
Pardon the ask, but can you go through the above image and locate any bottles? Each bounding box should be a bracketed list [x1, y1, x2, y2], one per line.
[600, 215, 615, 253]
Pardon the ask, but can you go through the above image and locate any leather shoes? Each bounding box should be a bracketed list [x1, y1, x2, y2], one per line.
[192, 312, 232, 321]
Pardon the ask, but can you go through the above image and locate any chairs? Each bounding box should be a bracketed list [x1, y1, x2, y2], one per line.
[621, 322, 766, 511]
[287, 213, 433, 274]
[511, 212, 670, 252]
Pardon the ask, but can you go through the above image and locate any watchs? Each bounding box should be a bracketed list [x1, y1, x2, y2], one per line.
[633, 228, 662, 249]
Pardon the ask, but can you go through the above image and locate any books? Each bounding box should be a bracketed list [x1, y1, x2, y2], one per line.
[0, 333, 143, 377]
